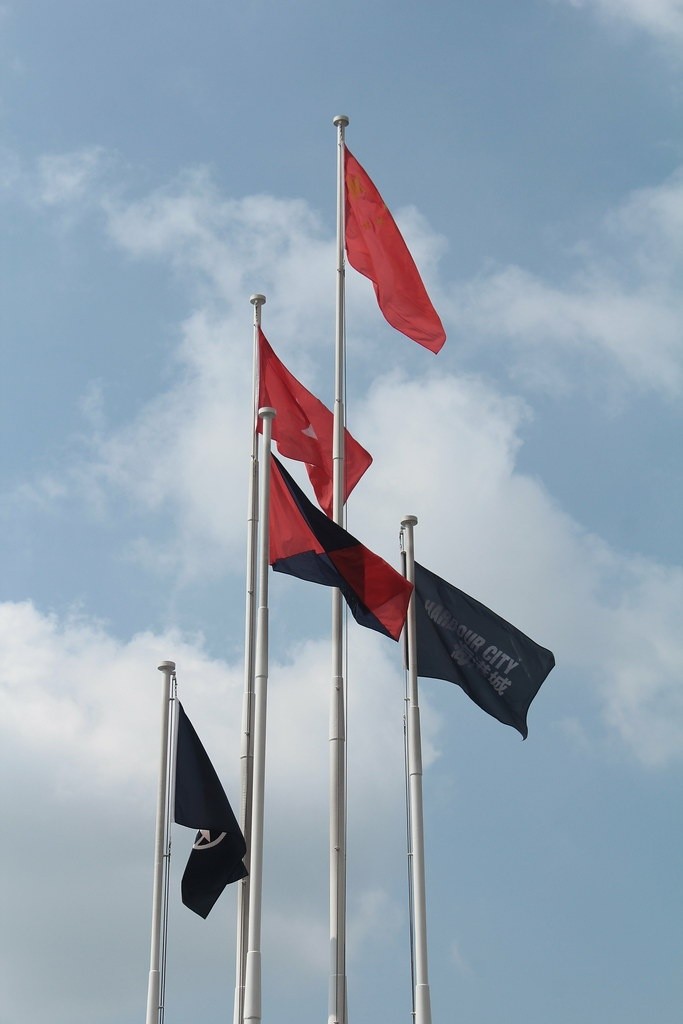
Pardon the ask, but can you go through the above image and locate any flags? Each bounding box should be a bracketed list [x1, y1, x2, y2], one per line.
[405, 560, 556, 740]
[268, 451, 414, 642]
[254, 325, 373, 521]
[344, 142, 446, 354]
[169, 698, 250, 921]
